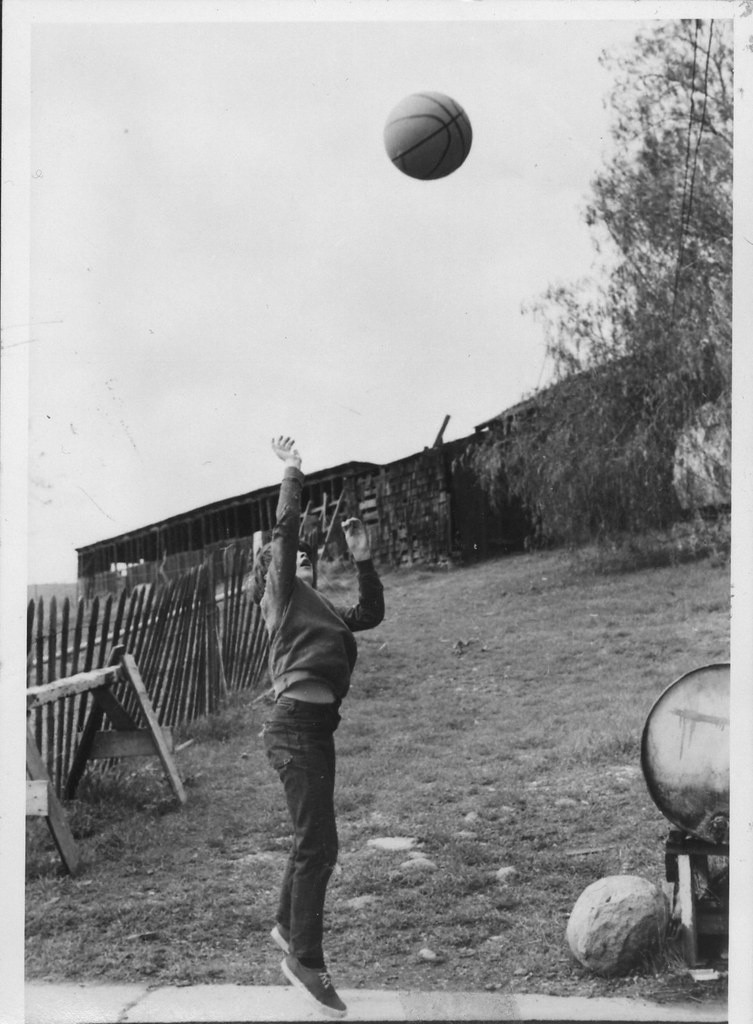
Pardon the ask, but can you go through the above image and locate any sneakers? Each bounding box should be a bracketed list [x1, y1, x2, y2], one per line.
[271, 923, 292, 956]
[280, 954, 347, 1018]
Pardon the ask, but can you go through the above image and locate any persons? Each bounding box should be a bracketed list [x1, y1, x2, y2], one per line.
[245, 437, 384, 1018]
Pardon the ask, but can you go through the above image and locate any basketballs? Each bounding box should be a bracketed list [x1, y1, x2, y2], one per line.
[382, 91, 473, 181]
